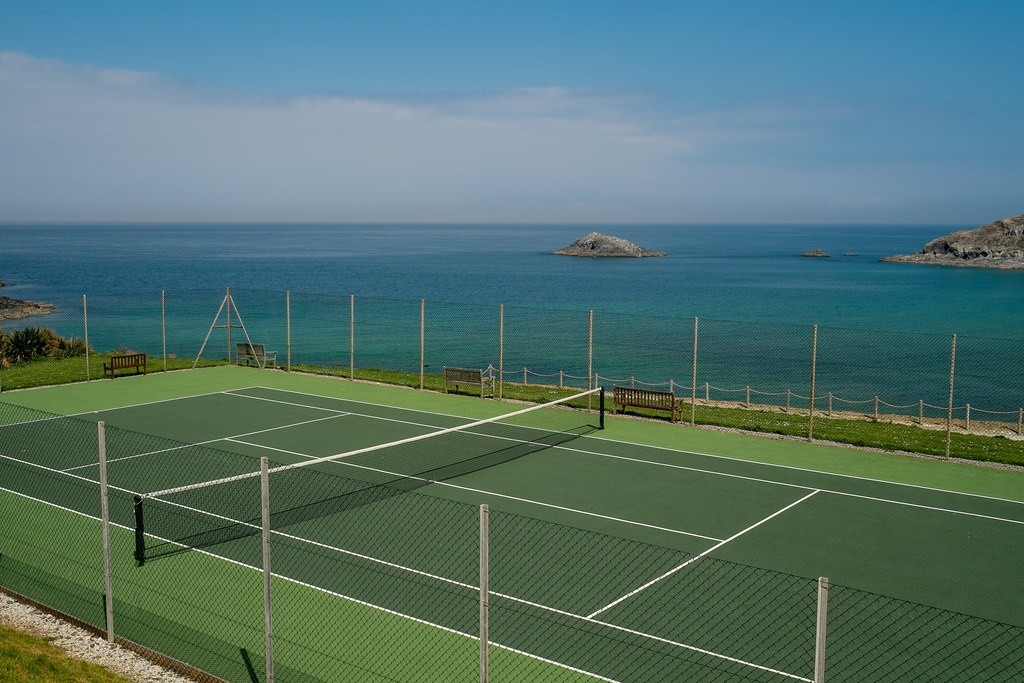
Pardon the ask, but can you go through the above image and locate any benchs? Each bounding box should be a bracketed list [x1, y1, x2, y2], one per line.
[443, 366, 496, 399]
[613, 386, 684, 423]
[236, 342, 277, 370]
[103, 353, 147, 379]
